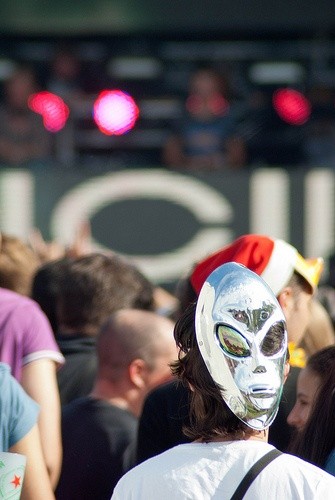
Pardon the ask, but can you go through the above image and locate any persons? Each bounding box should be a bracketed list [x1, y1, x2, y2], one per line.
[0, 54, 335, 500]
[109, 260, 335, 500]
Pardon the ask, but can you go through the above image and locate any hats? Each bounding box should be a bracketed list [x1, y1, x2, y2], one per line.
[190, 234, 298, 298]
